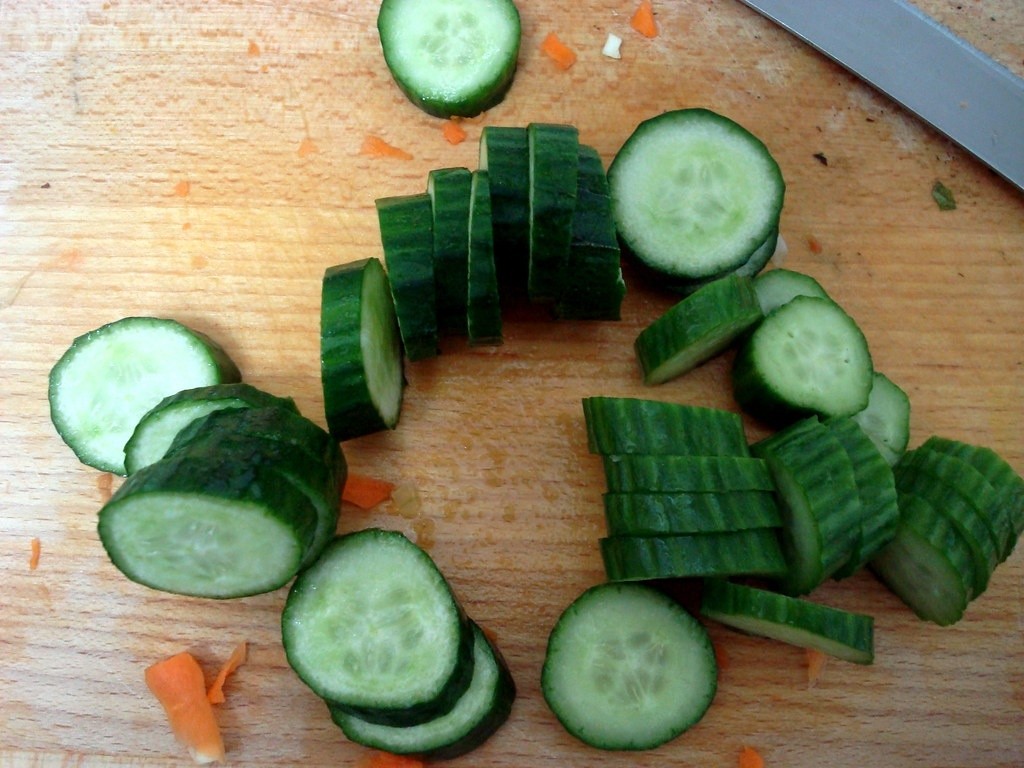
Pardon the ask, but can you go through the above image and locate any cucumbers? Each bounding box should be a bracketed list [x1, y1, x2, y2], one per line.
[50, 0, 1024, 759]
